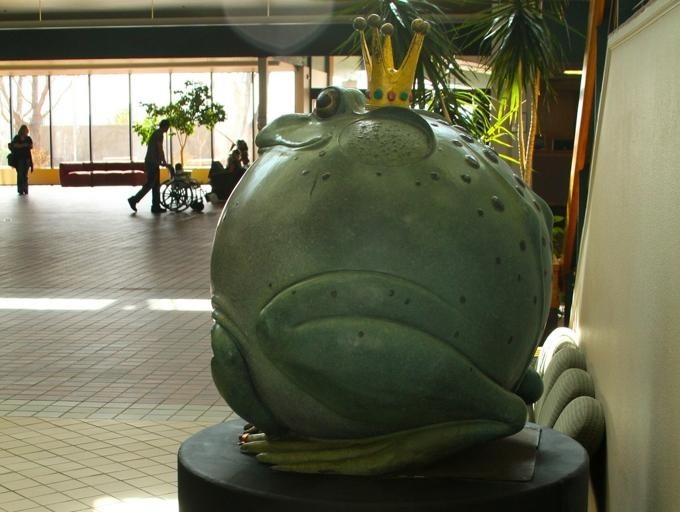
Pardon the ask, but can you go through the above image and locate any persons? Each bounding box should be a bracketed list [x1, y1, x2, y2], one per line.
[228, 149, 245, 172]
[8, 124, 33, 196]
[127, 119, 168, 214]
[175, 162, 194, 183]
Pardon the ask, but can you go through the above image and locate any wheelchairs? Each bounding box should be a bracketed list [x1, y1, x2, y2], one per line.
[157, 160, 206, 214]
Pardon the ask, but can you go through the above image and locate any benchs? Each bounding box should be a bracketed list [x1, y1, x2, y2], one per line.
[59, 163, 148, 185]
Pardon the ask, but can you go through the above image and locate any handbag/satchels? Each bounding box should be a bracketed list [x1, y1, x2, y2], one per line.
[6, 153, 16, 168]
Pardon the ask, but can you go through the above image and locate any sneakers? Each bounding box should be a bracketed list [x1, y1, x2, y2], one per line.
[128, 196, 167, 214]
